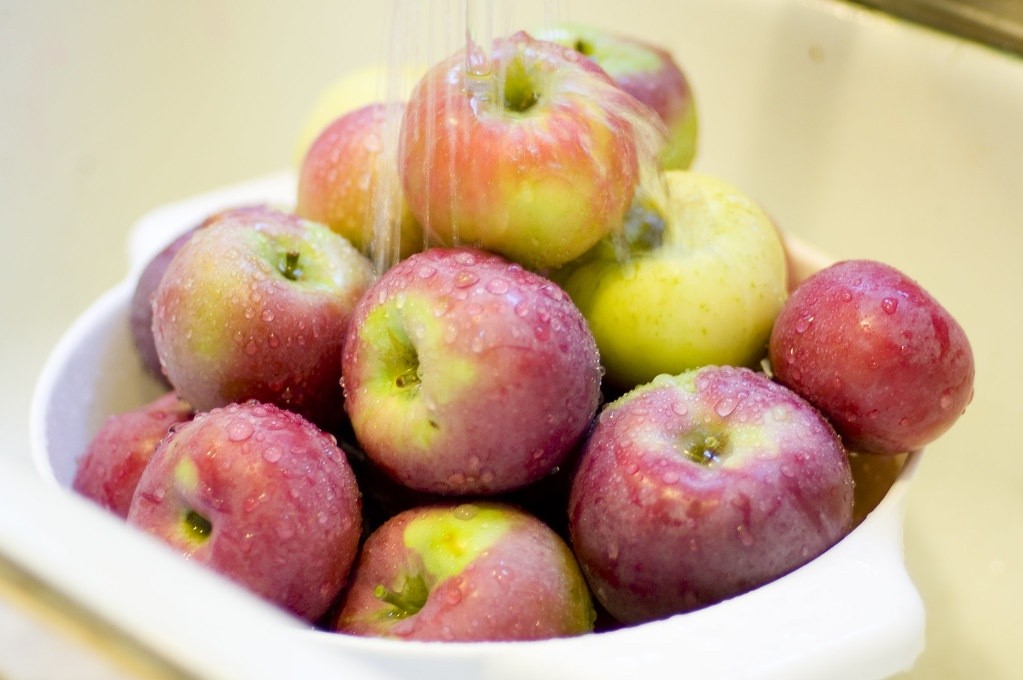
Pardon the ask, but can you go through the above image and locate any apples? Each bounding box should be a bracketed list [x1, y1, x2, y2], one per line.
[77, 25, 974, 642]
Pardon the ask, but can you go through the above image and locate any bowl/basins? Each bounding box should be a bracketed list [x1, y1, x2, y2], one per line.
[0, 174, 928, 680]
